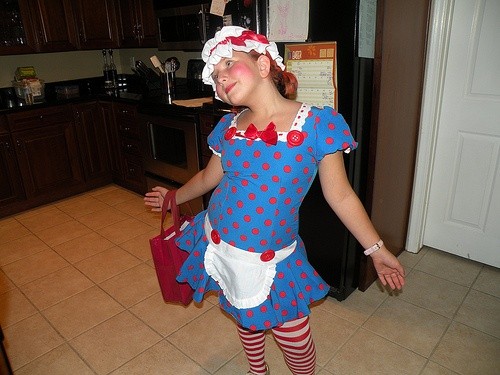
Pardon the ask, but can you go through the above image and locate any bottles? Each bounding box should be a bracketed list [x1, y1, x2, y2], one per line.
[101, 49, 118, 89]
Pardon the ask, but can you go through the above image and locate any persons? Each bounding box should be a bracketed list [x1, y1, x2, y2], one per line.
[144, 26, 404, 375]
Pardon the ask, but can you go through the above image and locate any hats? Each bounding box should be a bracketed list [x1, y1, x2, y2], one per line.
[201, 26, 286, 103]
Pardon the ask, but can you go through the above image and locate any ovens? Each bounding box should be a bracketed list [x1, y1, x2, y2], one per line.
[118, 105, 205, 216]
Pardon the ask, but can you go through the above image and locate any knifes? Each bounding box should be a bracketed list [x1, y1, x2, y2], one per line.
[130, 60, 149, 76]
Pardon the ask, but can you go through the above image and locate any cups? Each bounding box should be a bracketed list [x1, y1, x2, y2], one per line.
[159, 71, 175, 88]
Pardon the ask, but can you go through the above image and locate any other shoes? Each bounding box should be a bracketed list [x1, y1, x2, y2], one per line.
[246, 365, 269, 375]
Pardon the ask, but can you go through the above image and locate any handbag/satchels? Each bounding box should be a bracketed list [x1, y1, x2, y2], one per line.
[149, 189, 195, 306]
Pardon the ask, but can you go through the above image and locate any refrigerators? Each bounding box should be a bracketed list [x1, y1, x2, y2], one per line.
[215, 0, 377, 303]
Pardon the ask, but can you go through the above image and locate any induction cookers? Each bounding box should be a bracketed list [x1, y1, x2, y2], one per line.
[117, 58, 214, 115]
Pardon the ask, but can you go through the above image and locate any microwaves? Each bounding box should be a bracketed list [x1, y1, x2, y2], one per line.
[155, 4, 215, 51]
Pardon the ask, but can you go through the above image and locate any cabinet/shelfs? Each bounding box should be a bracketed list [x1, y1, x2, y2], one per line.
[0, 0, 375, 301]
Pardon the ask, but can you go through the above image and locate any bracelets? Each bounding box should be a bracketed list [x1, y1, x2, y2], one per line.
[364, 240, 384, 256]
